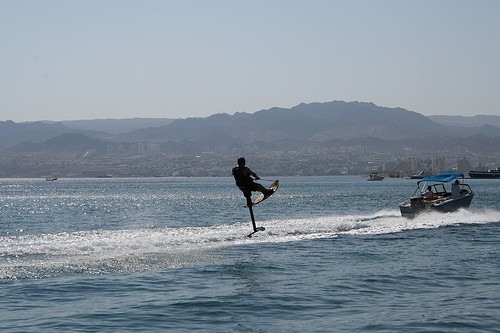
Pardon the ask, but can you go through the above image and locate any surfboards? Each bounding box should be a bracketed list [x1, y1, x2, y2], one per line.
[242, 179, 279, 208]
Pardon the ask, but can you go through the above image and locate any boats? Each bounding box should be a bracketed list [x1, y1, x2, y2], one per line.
[367, 173, 385, 181]
[468, 167, 500, 178]
[411, 171, 425, 179]
[398, 172, 475, 218]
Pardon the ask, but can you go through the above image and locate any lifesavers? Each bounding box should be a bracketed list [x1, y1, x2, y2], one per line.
[423, 195, 438, 200]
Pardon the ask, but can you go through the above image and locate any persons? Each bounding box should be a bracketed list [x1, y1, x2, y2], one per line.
[451, 180, 466, 198]
[232, 157, 274, 206]
[426, 186, 433, 196]
